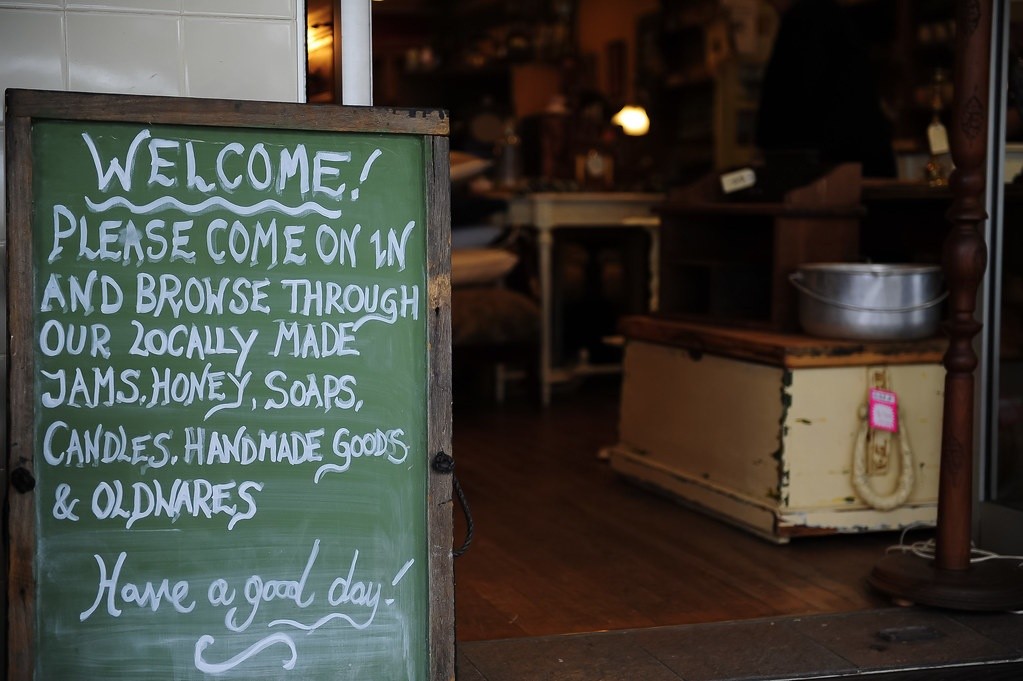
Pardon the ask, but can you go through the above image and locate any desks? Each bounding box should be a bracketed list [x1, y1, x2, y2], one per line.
[494, 192, 668, 411]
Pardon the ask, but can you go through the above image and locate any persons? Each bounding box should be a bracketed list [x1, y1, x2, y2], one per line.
[752, 0, 900, 205]
[541, 88, 652, 299]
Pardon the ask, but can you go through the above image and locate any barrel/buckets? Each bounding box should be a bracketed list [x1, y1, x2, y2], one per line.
[788, 264, 948, 339]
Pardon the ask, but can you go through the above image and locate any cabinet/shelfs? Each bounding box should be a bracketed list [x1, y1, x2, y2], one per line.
[650, 203, 867, 333]
[609, 316, 950, 545]
